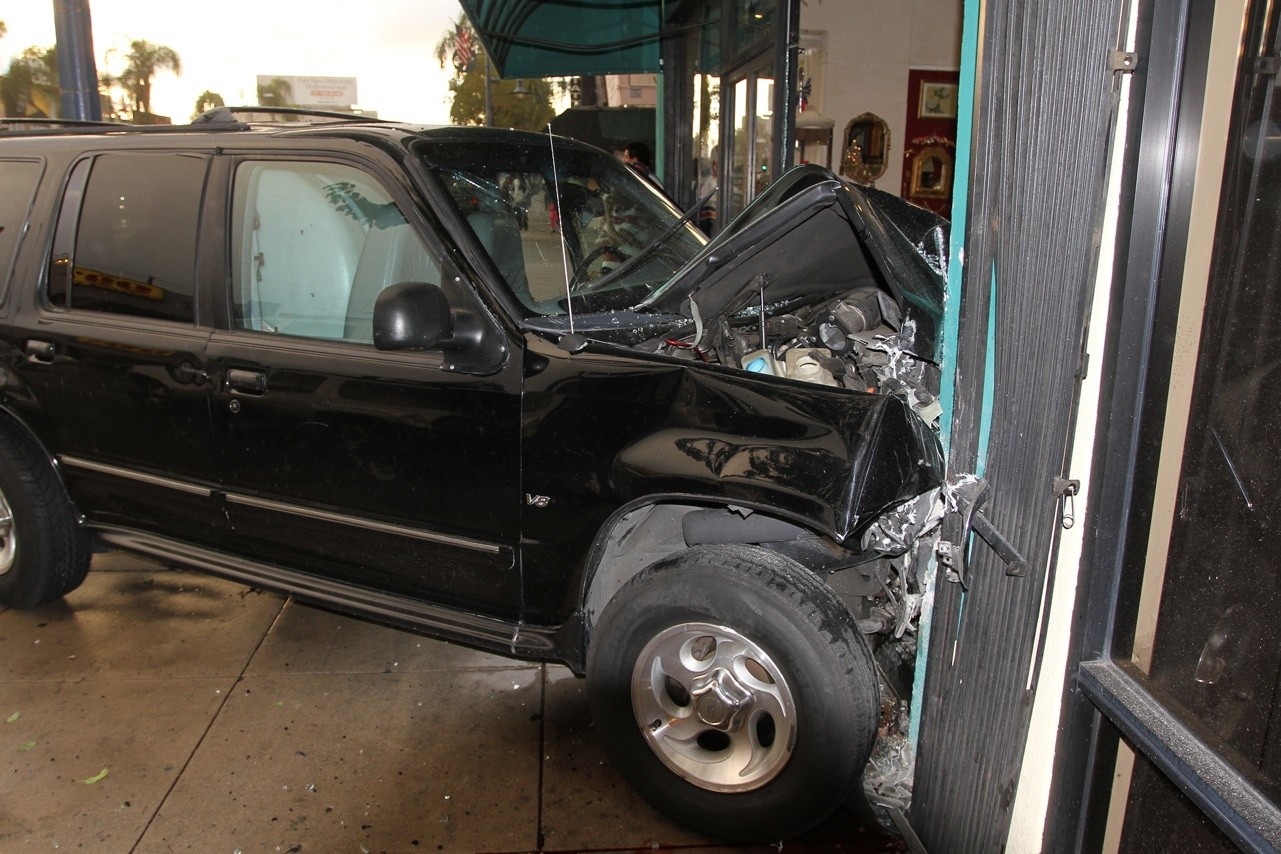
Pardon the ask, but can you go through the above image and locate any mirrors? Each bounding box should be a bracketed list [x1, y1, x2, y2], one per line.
[841, 111, 891, 185]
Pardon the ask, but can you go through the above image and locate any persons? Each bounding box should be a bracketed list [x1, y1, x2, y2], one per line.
[623, 141, 663, 190]
[542, 183, 551, 211]
[547, 200, 560, 234]
[502, 172, 515, 190]
[594, 189, 651, 278]
[511, 171, 531, 231]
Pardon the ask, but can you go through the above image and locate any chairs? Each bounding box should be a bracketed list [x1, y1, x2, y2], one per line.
[344, 202, 444, 342]
[467, 184, 566, 313]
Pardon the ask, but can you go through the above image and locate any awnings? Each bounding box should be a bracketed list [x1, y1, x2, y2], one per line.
[459, 0, 661, 79]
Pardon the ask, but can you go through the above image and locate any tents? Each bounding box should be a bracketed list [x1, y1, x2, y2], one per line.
[540, 105, 656, 167]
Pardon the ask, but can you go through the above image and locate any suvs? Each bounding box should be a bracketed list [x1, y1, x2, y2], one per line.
[0, 106, 951, 846]
